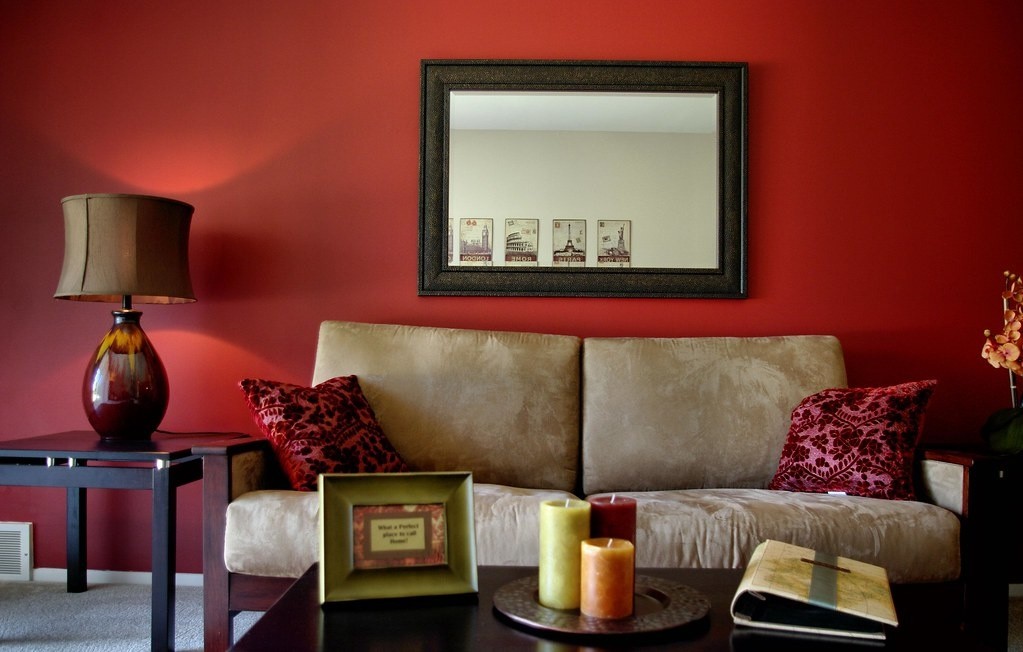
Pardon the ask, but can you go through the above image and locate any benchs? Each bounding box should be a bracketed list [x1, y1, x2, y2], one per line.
[191, 320, 1008, 652]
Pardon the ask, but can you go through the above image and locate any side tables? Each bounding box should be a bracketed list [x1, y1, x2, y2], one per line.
[0, 430, 251, 652]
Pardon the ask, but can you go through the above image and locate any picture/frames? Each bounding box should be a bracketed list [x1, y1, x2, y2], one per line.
[420, 59, 749, 299]
[317, 470, 478, 605]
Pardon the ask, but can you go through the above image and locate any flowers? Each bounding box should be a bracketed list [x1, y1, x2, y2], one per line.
[981, 271, 1023, 407]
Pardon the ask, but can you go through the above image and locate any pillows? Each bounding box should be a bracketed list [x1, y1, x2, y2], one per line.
[238, 374, 412, 491]
[768, 380, 942, 502]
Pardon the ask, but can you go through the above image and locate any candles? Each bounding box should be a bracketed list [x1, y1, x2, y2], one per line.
[581, 538, 634, 619]
[537, 499, 592, 609]
[591, 494, 637, 594]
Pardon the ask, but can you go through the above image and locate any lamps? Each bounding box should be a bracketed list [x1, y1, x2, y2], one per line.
[53, 193, 197, 443]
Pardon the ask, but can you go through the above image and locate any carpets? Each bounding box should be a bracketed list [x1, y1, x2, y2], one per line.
[0, 580, 266, 652]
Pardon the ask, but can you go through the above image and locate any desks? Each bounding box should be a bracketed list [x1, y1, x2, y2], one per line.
[230, 563, 998, 652]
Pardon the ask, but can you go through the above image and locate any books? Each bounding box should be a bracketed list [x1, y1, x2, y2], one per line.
[729, 540, 898, 641]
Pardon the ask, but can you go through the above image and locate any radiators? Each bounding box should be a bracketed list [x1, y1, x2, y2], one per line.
[0, 521, 33, 580]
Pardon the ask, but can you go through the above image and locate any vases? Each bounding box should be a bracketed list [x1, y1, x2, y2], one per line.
[982, 408, 1023, 457]
